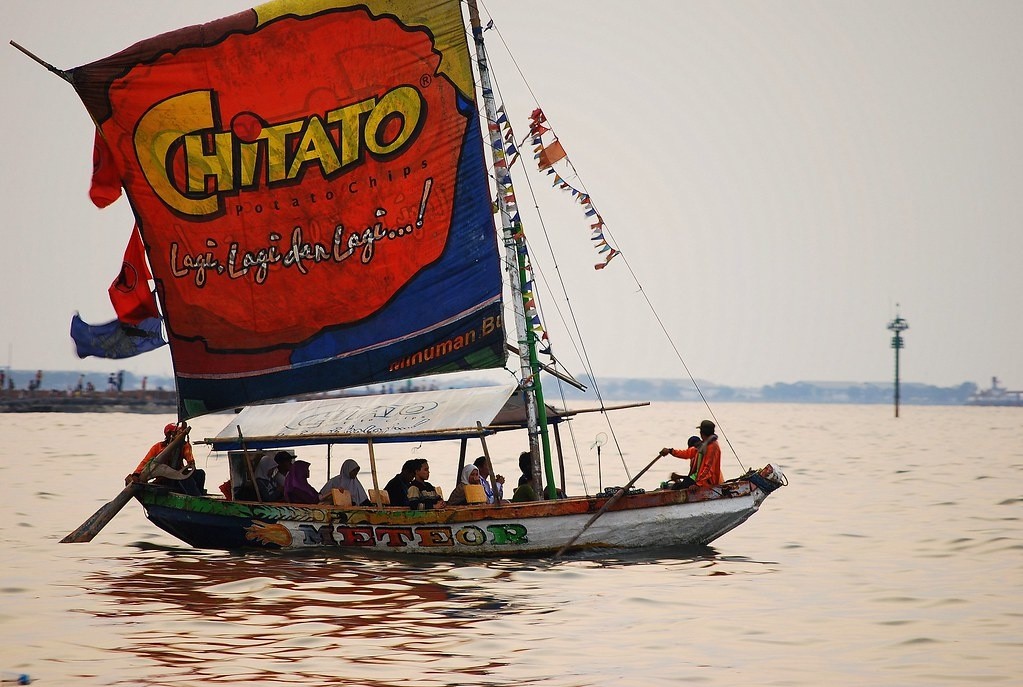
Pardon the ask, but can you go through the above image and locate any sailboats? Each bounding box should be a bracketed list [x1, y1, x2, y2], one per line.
[123, 0, 787, 558]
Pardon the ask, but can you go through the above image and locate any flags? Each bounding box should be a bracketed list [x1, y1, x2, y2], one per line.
[552, 174, 589, 204]
[528, 107, 550, 153]
[522, 280, 551, 356]
[66, 0, 507, 421]
[585, 203, 620, 270]
[534, 154, 557, 175]
[539, 141, 566, 171]
[488, 102, 518, 212]
[71, 292, 166, 359]
[520, 263, 531, 272]
[511, 213, 527, 256]
[109, 224, 160, 325]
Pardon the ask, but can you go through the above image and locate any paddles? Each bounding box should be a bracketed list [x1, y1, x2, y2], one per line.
[58, 428, 192, 543]
[554, 453, 662, 557]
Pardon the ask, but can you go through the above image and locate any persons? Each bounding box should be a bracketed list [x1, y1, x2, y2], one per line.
[447, 456, 505, 504]
[662, 420, 721, 493]
[108, 370, 125, 392]
[320, 459, 369, 507]
[518, 451, 533, 490]
[249, 449, 298, 502]
[77, 374, 94, 392]
[285, 461, 323, 505]
[142, 377, 147, 390]
[132, 424, 205, 489]
[383, 458, 446, 509]
[0, 370, 42, 390]
[379, 379, 442, 394]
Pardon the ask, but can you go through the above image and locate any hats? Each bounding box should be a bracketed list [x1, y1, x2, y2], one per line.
[164, 424, 181, 435]
[274, 451, 297, 462]
[696, 420, 715, 430]
